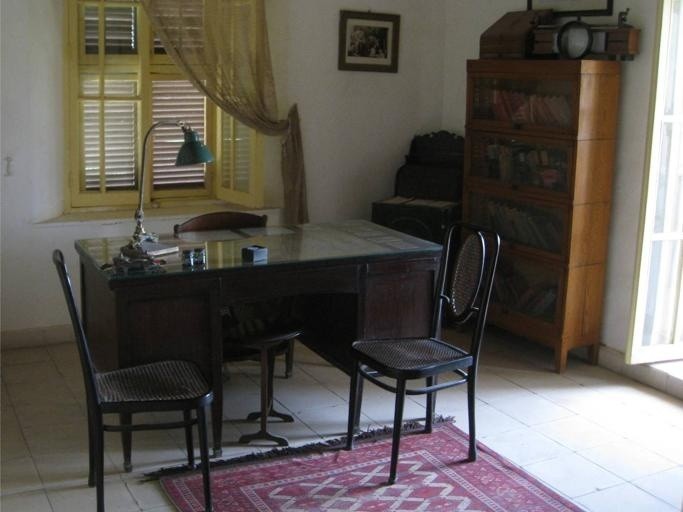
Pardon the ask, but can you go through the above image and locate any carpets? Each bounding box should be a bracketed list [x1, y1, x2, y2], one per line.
[139, 415, 587, 512]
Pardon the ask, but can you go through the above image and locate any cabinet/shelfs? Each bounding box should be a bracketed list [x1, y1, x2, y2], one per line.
[370, 197, 457, 255]
[458, 55, 623, 376]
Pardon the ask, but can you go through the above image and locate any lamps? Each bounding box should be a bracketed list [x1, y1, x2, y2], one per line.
[126, 120, 216, 248]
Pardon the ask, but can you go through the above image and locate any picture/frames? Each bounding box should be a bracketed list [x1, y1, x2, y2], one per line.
[337, 9, 400, 74]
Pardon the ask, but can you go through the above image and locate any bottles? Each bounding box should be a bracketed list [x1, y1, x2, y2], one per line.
[180, 246, 207, 268]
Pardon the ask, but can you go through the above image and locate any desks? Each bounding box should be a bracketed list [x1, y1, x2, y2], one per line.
[231, 325, 301, 446]
[73, 219, 446, 383]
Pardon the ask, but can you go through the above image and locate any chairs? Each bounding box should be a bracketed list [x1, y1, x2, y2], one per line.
[173, 211, 302, 378]
[51, 248, 216, 512]
[343, 222, 503, 485]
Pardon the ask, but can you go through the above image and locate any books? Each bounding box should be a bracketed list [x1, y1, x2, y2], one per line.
[472, 85, 576, 325]
